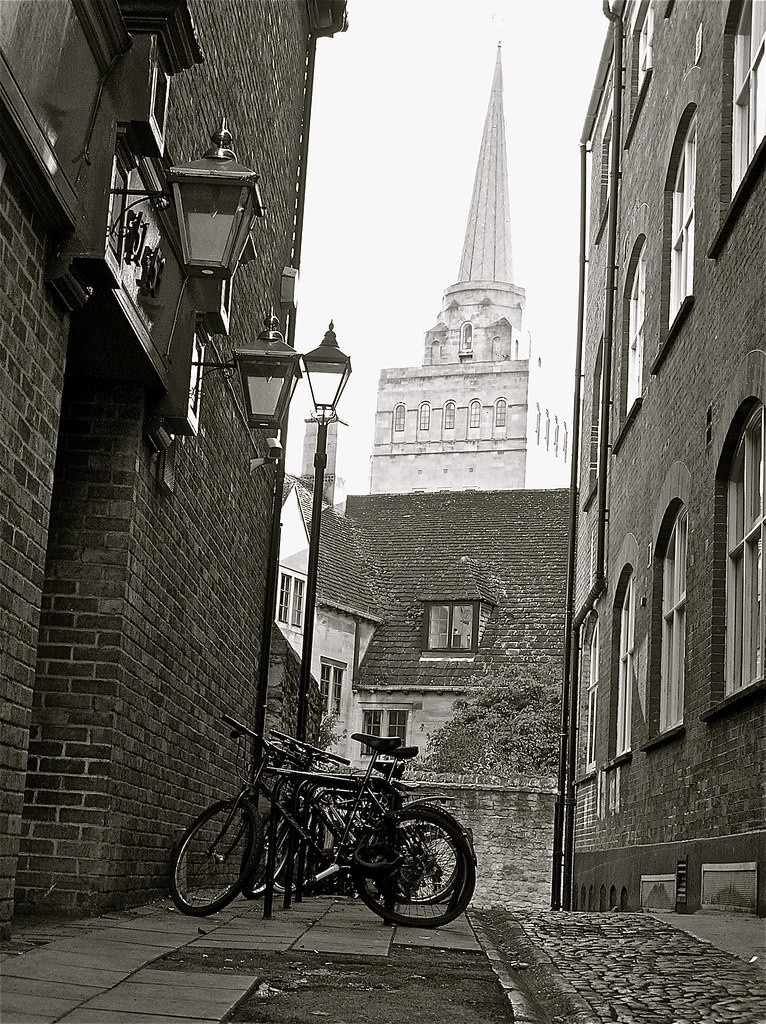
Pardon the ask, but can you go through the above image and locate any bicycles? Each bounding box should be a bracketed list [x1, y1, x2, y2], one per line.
[169, 715, 477, 930]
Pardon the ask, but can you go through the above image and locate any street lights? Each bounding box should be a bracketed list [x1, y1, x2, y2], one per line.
[295, 320, 353, 740]
